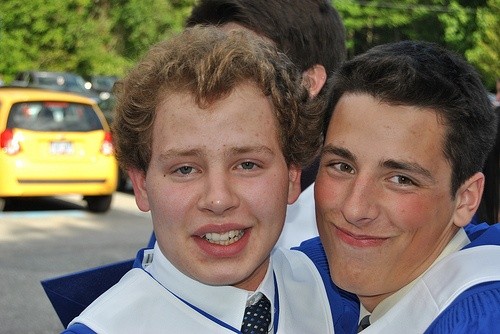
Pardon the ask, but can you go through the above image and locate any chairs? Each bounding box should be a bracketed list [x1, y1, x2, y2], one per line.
[35, 108, 52, 121]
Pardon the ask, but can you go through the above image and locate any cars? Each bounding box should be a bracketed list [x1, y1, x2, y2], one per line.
[11, 70, 136, 194]
[0, 85, 119, 213]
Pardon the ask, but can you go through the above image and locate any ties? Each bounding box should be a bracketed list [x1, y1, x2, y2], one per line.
[241, 299, 271, 334]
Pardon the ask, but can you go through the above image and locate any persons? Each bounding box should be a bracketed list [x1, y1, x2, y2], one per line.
[473, 106, 500, 226]
[184, 0, 350, 251]
[310, 39, 500, 333]
[62, 22, 360, 333]
